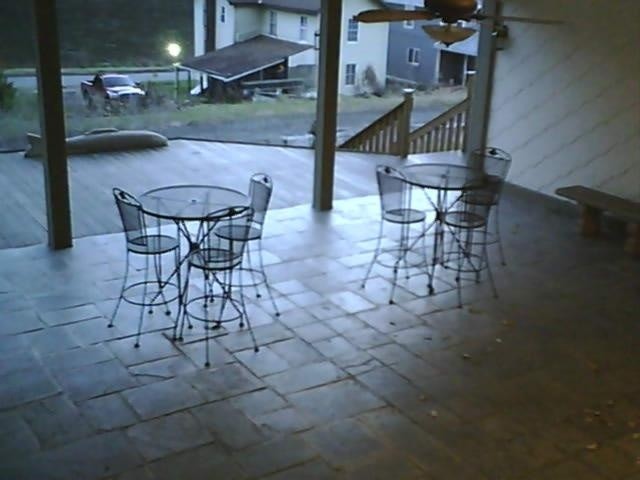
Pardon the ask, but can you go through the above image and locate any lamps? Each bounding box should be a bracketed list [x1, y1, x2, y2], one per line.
[420, 18, 478, 48]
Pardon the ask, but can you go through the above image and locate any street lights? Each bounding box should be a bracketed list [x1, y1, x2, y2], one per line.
[166, 42, 183, 100]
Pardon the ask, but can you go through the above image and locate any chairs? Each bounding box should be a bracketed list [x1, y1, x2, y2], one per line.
[107, 185, 193, 349]
[203, 172, 282, 329]
[441, 146, 512, 285]
[178, 204, 260, 369]
[430, 178, 498, 309]
[361, 163, 432, 305]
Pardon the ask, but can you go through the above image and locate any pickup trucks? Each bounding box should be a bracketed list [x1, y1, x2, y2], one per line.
[78, 74, 149, 112]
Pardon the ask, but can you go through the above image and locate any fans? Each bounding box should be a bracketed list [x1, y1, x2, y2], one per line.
[349, 0, 570, 24]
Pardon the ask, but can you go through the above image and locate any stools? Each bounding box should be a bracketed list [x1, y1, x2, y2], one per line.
[554, 183, 639, 252]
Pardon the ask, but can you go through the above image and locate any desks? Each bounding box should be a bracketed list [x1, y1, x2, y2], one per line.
[398, 163, 489, 290]
[138, 183, 245, 343]
